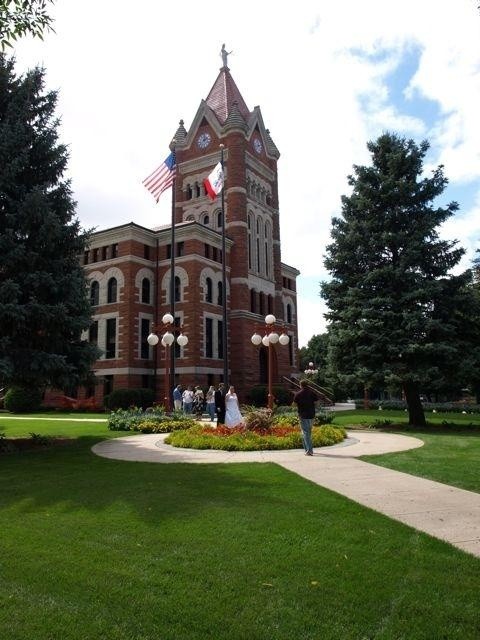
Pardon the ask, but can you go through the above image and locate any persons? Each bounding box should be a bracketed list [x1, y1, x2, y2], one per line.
[206, 385, 216, 423]
[192, 385, 205, 418]
[214, 382, 226, 428]
[225, 384, 244, 430]
[202, 159, 225, 202]
[291, 378, 319, 458]
[140, 149, 178, 206]
[181, 383, 194, 415]
[172, 384, 182, 414]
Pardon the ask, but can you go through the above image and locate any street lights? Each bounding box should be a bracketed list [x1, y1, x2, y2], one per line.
[304, 362, 319, 380]
[251, 315, 290, 417]
[147, 312, 189, 417]
